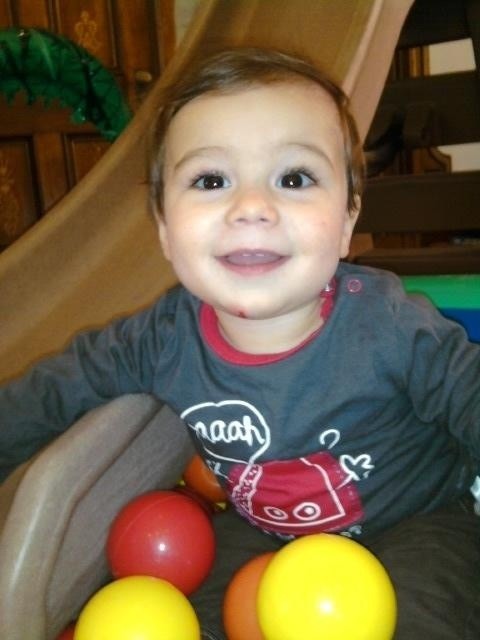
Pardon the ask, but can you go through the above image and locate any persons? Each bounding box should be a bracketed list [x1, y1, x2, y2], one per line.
[0, 46, 480, 640]
